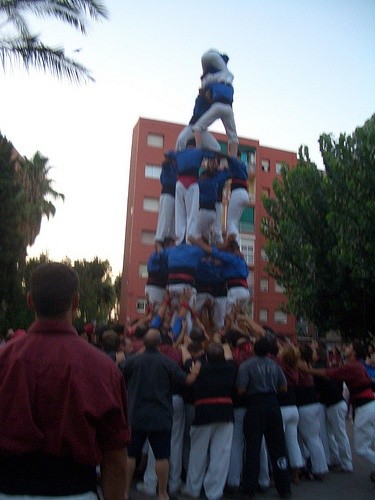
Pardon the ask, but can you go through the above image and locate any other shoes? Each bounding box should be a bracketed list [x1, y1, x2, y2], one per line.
[302, 473, 324, 481]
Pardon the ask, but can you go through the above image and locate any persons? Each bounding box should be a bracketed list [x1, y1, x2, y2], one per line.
[77, 49, 375, 500]
[0, 262, 131, 500]
